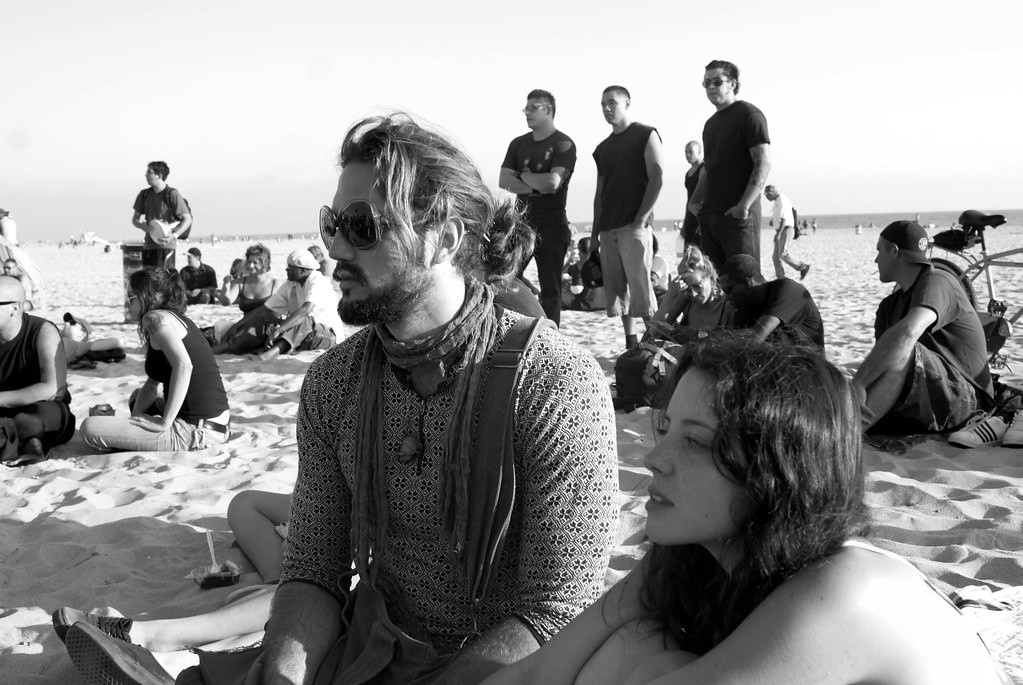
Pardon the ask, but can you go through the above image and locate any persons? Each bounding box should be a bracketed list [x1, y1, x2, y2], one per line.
[0, 275, 76, 466]
[688, 60, 771, 288]
[248, 116, 621, 685]
[53, 488, 295, 685]
[80, 267, 231, 452]
[680, 141, 706, 255]
[589, 86, 662, 349]
[132, 161, 192, 270]
[180, 243, 346, 362]
[853, 220, 995, 452]
[59, 313, 118, 367]
[764, 185, 811, 281]
[639, 246, 824, 349]
[485, 340, 1010, 685]
[798, 217, 817, 235]
[560, 221, 669, 312]
[0, 207, 34, 311]
[499, 89, 576, 328]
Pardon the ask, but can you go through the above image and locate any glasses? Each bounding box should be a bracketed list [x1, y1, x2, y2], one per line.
[319, 199, 491, 253]
[147, 170, 155, 175]
[66, 319, 76, 325]
[702, 78, 731, 88]
[124, 292, 160, 306]
[523, 104, 552, 114]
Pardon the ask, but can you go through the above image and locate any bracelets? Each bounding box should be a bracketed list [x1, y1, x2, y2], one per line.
[697, 328, 708, 341]
[173, 233, 179, 239]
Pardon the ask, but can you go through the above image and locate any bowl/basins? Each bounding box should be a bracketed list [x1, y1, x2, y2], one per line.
[193, 560, 240, 589]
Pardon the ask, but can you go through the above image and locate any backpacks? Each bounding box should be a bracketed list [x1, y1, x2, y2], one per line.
[143, 187, 193, 240]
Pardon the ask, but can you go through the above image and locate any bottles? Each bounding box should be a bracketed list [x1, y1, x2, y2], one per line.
[88, 404, 116, 416]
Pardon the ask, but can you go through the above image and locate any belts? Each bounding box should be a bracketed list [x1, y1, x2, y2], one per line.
[184, 416, 230, 433]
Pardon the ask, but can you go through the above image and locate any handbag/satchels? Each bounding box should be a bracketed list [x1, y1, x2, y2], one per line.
[612, 340, 683, 414]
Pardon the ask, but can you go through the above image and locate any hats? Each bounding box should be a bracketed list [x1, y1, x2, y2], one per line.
[287, 249, 320, 269]
[63, 313, 92, 341]
[880, 221, 932, 266]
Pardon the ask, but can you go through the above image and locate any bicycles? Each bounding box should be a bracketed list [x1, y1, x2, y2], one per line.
[892, 210, 1023, 371]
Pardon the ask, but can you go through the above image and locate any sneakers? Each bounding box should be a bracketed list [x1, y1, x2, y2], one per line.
[65, 620, 176, 685]
[1002, 409, 1023, 445]
[52, 607, 133, 648]
[948, 409, 1009, 449]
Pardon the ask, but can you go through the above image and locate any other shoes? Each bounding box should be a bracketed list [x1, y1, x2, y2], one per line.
[801, 265, 810, 280]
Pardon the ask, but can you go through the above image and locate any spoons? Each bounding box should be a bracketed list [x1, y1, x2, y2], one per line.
[206, 529, 221, 574]
